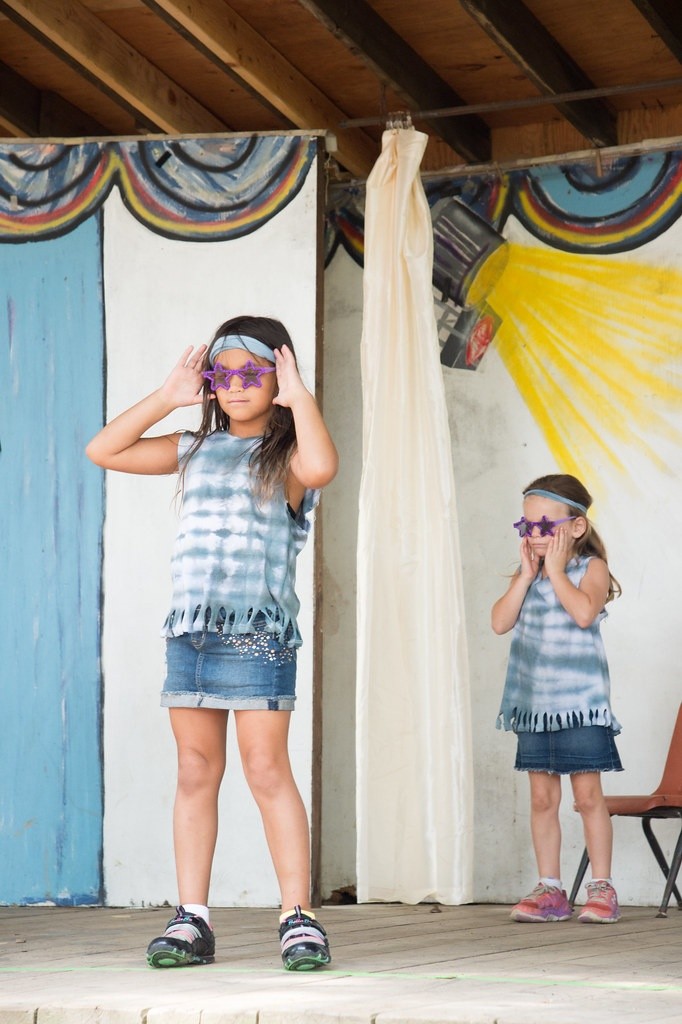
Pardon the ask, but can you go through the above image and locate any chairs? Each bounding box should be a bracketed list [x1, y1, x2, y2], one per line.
[569, 701, 682, 919]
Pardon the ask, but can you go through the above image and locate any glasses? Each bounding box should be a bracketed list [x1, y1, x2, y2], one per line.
[513, 514, 577, 537]
[202, 360, 276, 390]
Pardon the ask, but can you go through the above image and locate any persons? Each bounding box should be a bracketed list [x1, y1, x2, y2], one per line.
[492, 473, 625, 925]
[85, 315, 340, 970]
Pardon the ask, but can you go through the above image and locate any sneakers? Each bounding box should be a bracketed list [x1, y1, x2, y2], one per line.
[509, 882, 573, 921]
[278, 905, 331, 971]
[577, 880, 622, 922]
[146, 906, 215, 967]
